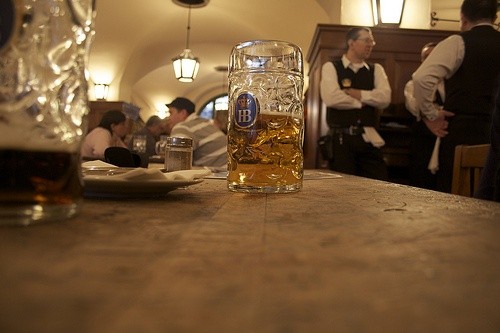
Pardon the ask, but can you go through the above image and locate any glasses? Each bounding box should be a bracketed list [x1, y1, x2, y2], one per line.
[353, 38, 376, 47]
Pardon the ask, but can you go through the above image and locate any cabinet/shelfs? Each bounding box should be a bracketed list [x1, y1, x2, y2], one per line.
[88, 100, 142, 138]
[305, 20, 459, 181]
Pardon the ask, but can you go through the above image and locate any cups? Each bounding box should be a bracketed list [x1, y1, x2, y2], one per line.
[0, 0, 97, 226]
[226, 40, 306, 193]
[165, 137, 193, 190]
[133, 135, 146, 153]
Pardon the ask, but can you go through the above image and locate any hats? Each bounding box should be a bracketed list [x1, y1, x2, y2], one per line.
[166, 97, 195, 114]
[104, 147, 141, 168]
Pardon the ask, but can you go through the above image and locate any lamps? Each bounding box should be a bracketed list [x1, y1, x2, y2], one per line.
[169, 0, 211, 84]
[369, 0, 406, 29]
[93, 82, 110, 100]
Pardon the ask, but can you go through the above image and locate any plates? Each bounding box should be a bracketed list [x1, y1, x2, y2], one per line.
[80, 165, 205, 197]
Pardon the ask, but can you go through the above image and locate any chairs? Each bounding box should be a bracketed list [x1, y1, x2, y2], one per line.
[451, 143, 491, 198]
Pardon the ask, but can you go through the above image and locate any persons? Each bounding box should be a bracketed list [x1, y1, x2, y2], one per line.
[82, 109, 128, 161]
[411, 0, 500, 202]
[320, 27, 392, 153]
[165, 98, 229, 168]
[129, 116, 162, 166]
[403, 42, 439, 190]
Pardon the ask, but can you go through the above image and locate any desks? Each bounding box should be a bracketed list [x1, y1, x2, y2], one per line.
[0, 165, 500, 333]
